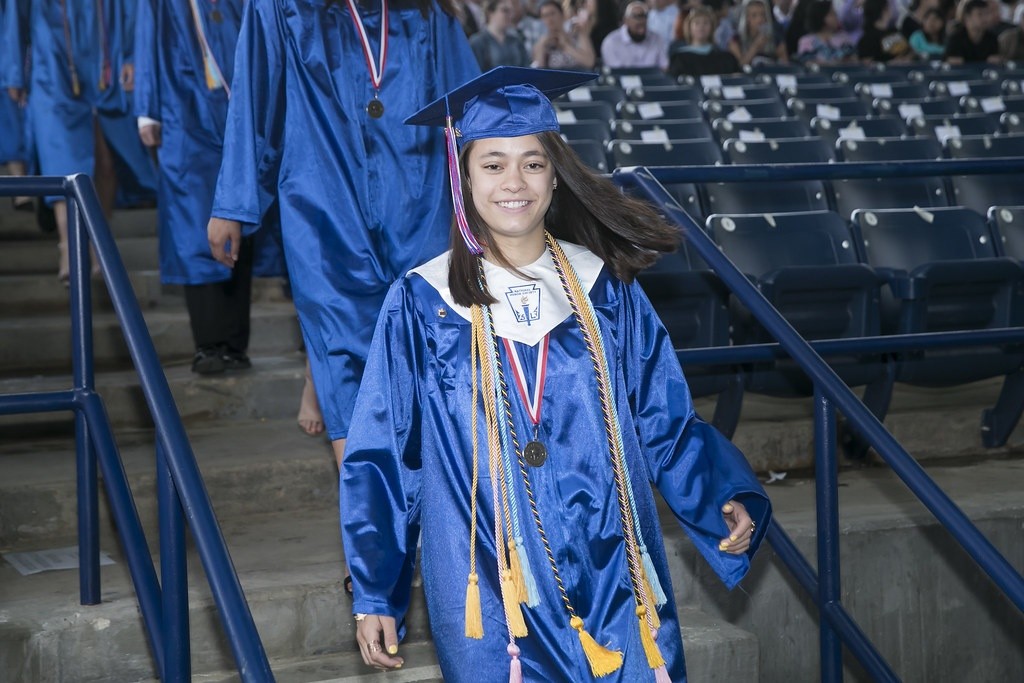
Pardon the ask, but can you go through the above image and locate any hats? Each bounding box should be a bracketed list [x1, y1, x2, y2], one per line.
[401, 66, 599, 253]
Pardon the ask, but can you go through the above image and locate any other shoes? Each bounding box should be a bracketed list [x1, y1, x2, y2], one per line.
[58, 267, 70, 288]
[12, 195, 32, 210]
[192, 349, 224, 375]
[298, 378, 324, 436]
[224, 352, 252, 371]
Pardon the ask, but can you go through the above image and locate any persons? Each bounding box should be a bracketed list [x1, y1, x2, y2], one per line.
[0, 0, 1024, 595]
[339, 65, 772, 683]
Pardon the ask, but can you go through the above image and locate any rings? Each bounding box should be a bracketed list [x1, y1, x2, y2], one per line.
[751, 520, 756, 532]
[367, 640, 382, 653]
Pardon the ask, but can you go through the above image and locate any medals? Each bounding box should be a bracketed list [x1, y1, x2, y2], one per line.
[523, 438, 547, 467]
[210, 10, 224, 24]
[365, 99, 384, 119]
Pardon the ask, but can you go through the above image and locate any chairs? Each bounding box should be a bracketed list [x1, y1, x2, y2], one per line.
[551, 66, 947, 120]
[958, 94, 1024, 113]
[835, 135, 944, 165]
[947, 132, 1024, 159]
[566, 139, 608, 174]
[560, 120, 611, 142]
[605, 138, 725, 174]
[851, 206, 1024, 450]
[722, 135, 839, 167]
[828, 178, 949, 222]
[945, 60, 1024, 103]
[635, 234, 744, 446]
[617, 182, 702, 224]
[811, 115, 908, 139]
[906, 113, 994, 139]
[712, 115, 811, 144]
[611, 118, 714, 140]
[701, 176, 831, 218]
[947, 170, 1024, 210]
[705, 210, 893, 456]
